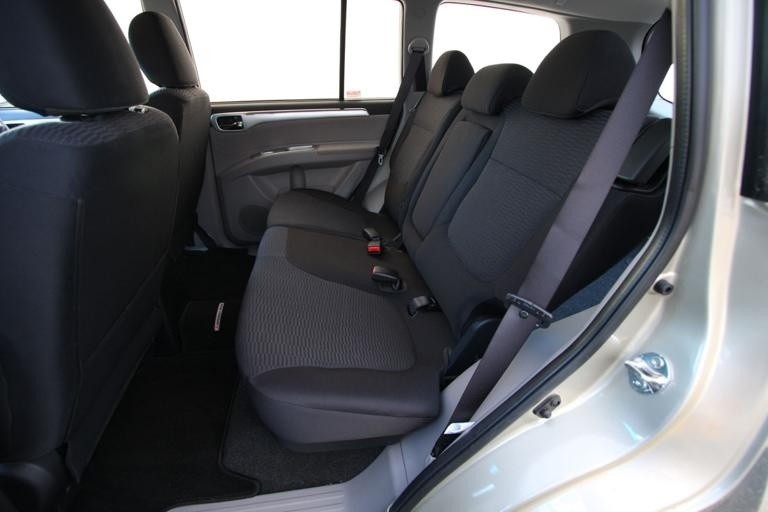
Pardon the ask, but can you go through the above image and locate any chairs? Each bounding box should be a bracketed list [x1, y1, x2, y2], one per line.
[1, 0, 179, 508]
[121, 10, 210, 266]
[233, 28, 635, 445]
